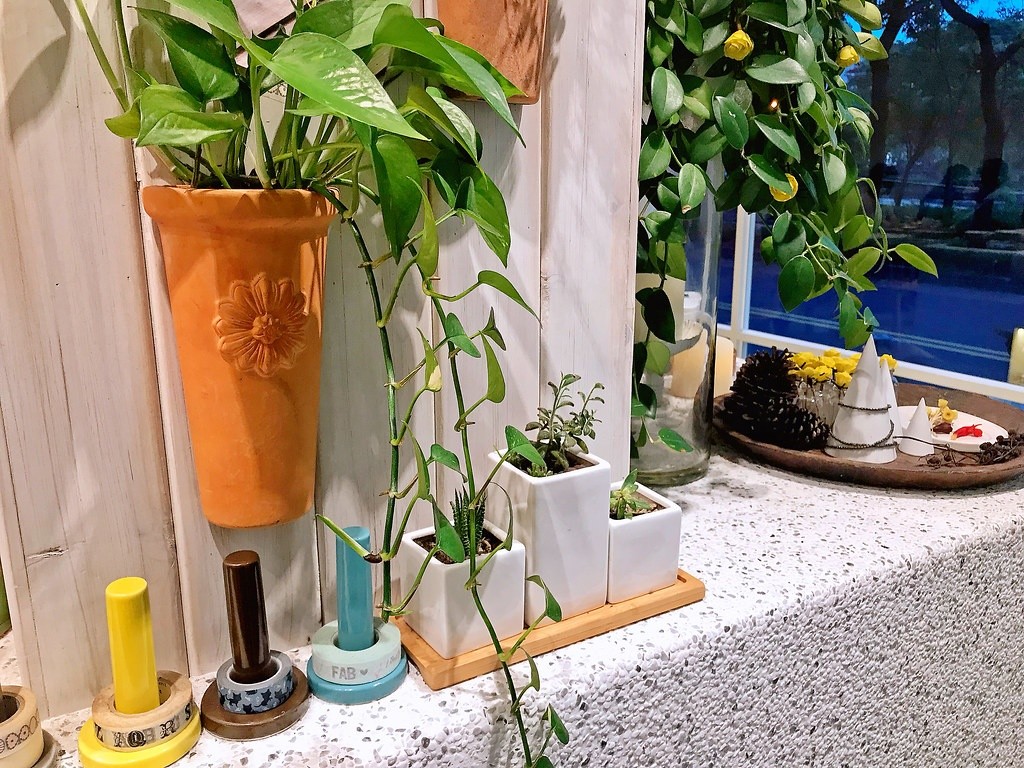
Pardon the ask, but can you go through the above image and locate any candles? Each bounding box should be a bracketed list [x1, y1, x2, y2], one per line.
[668, 327, 735, 399]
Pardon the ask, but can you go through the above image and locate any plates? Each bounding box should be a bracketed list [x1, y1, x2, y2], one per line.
[898, 406, 1009, 452]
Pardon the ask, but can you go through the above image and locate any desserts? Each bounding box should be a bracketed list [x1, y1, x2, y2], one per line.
[785, 349, 896, 386]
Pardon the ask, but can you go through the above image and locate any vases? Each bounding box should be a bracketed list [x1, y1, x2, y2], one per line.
[628, 103, 726, 487]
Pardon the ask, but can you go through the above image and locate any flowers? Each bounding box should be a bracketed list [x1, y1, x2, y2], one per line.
[784, 349, 896, 406]
[629, 0, 938, 461]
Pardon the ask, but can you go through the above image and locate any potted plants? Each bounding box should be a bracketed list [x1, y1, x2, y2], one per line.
[73, 0, 571, 768]
[402, 372, 682, 659]
